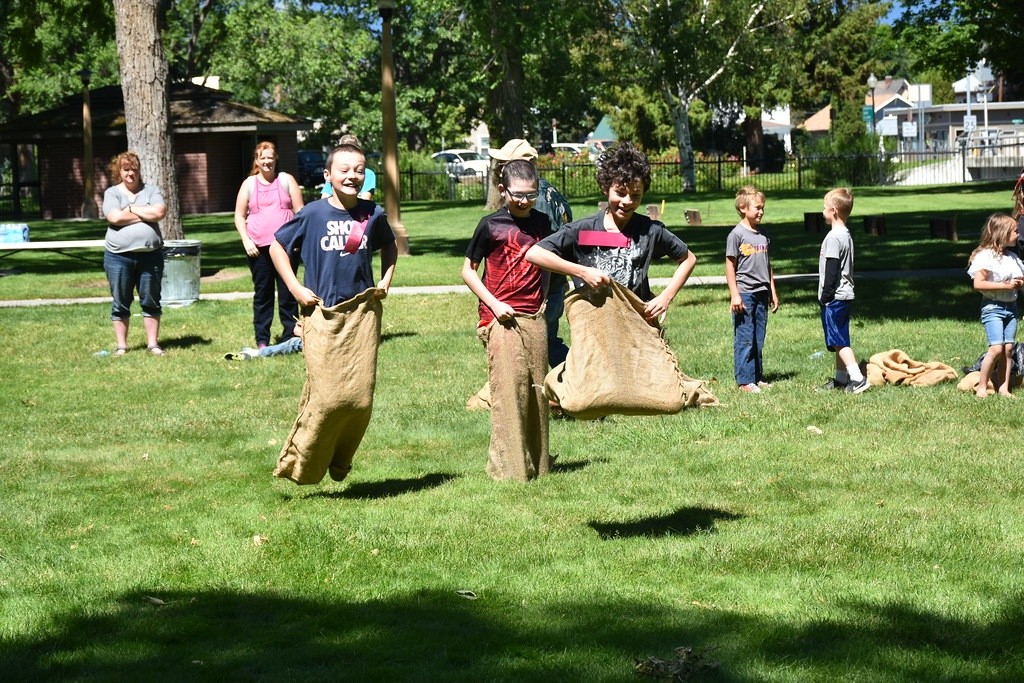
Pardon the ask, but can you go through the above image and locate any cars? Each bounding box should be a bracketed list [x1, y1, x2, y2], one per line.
[430, 148, 490, 182]
[297, 149, 328, 188]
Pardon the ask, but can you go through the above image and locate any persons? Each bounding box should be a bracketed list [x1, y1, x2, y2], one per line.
[488, 139, 573, 369]
[102, 153, 167, 355]
[1007, 171, 1024, 316]
[525, 141, 720, 420]
[321, 135, 376, 200]
[224, 321, 303, 360]
[966, 212, 1024, 397]
[726, 185, 778, 393]
[462, 159, 561, 482]
[234, 142, 304, 347]
[268, 145, 398, 485]
[818, 188, 870, 392]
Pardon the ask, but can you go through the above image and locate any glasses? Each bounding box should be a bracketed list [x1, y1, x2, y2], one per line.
[498, 160, 507, 170]
[504, 185, 539, 204]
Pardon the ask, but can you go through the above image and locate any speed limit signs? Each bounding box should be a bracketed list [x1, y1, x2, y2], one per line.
[964, 115, 977, 132]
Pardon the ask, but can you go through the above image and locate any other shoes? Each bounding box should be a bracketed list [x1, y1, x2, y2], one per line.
[224, 353, 246, 362]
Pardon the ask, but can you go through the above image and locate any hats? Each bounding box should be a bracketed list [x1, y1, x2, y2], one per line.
[339, 134, 360, 149]
[488, 138, 538, 161]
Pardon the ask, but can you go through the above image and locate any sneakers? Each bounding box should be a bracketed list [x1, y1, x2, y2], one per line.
[757, 381, 771, 389]
[739, 383, 763, 394]
[842, 376, 870, 397]
[815, 377, 848, 393]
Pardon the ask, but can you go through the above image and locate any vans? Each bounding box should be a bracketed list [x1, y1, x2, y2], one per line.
[474, 134, 492, 161]
[533, 138, 620, 164]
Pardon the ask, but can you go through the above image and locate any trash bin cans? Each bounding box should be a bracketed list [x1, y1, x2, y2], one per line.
[159, 240, 200, 307]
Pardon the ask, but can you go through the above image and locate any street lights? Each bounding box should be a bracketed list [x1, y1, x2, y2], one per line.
[868, 72, 879, 186]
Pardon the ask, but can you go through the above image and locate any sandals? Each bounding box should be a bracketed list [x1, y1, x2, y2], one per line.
[114, 347, 127, 359]
[146, 346, 166, 358]
